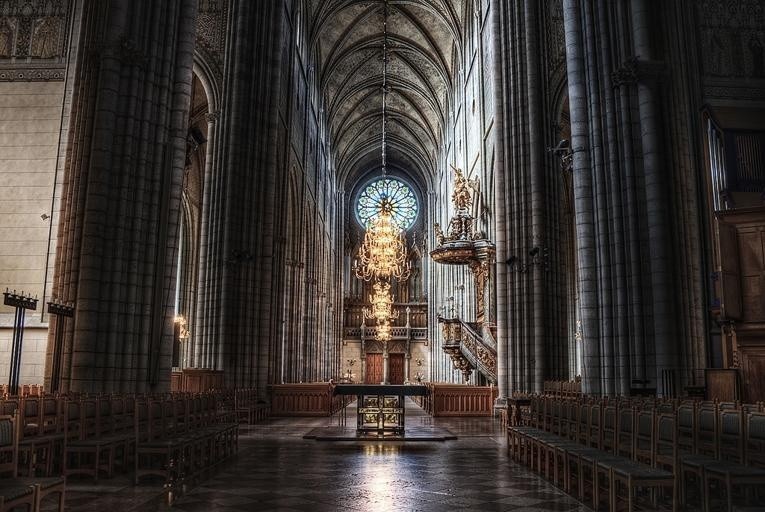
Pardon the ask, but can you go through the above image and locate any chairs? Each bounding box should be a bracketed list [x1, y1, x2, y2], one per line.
[505, 378, 765, 511]
[0, 380, 274, 511]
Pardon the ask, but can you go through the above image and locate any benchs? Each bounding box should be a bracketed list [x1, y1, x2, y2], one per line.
[274, 379, 360, 417]
[407, 381, 492, 418]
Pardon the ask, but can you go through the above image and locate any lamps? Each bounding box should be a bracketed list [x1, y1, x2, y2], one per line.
[350, 2, 423, 344]
